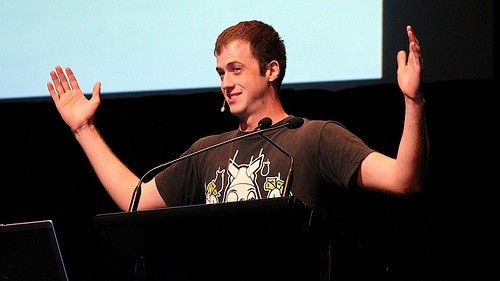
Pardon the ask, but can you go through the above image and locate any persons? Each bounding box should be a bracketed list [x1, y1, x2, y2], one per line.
[47, 20, 429, 213]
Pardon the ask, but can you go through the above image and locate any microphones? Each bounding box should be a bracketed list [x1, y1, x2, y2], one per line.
[128, 117, 304, 213]
[221, 101, 226, 112]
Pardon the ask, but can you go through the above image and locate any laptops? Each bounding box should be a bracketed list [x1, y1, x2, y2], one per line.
[0, 219, 68, 281]
